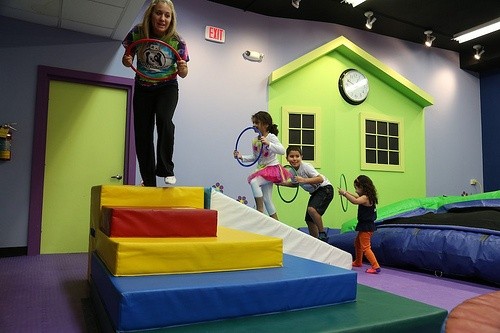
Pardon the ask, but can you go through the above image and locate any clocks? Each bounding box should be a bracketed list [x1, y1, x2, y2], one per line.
[338, 68, 370, 105]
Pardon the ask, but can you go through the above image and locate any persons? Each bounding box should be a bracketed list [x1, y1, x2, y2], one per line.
[283, 146, 334, 243]
[121, 0, 190, 187]
[233, 111, 286, 220]
[338, 175, 381, 273]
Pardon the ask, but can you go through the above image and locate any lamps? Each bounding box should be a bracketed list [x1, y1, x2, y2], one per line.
[291, 0, 500, 67]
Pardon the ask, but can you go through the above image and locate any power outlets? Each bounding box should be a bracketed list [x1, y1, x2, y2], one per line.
[470, 179, 476, 185]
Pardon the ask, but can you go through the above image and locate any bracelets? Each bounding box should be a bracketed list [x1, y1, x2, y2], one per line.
[343, 192, 346, 195]
